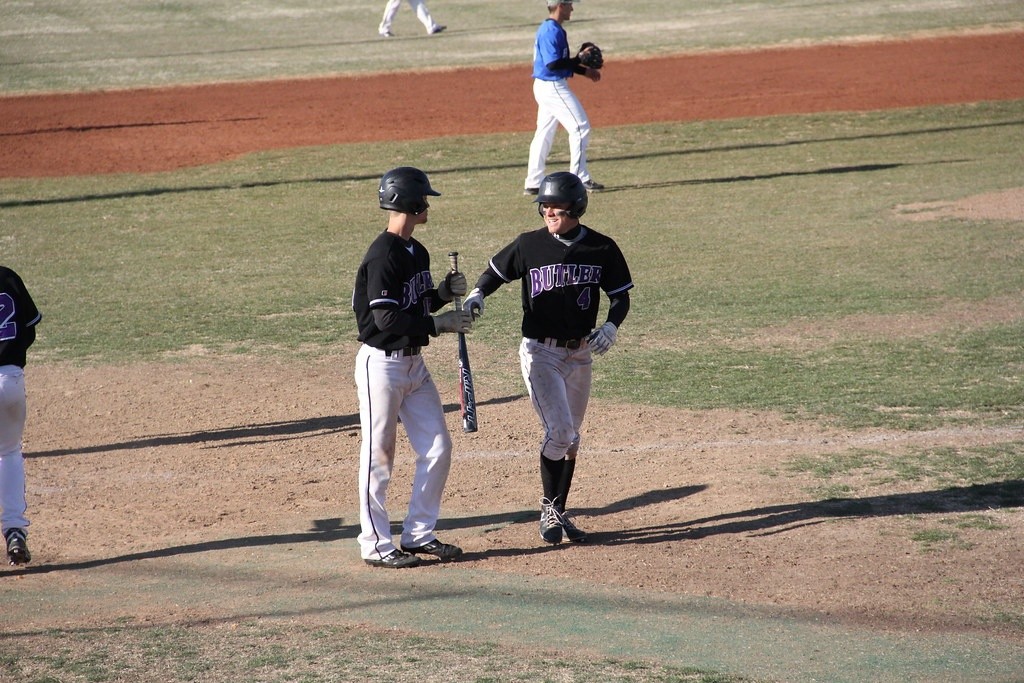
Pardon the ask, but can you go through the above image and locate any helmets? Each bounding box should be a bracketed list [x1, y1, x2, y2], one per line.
[378, 167, 442, 216]
[532, 171, 588, 219]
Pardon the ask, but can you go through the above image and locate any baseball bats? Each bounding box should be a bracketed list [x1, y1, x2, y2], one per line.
[448, 251, 478, 433]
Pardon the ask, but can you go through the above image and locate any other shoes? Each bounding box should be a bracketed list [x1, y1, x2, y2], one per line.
[432, 25, 447, 34]
[523, 188, 539, 195]
[584, 179, 605, 192]
[384, 31, 394, 37]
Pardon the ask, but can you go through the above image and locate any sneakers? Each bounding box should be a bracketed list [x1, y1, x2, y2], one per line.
[401, 538, 463, 562]
[539, 503, 588, 543]
[5, 528, 32, 565]
[364, 549, 421, 568]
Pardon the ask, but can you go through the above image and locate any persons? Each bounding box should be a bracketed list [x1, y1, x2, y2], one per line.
[351, 167, 474, 570]
[0, 266, 42, 565]
[378, 0, 447, 38]
[523, 0, 605, 195]
[463, 172, 634, 543]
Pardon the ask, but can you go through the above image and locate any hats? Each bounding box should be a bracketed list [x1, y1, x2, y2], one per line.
[548, 0, 579, 4]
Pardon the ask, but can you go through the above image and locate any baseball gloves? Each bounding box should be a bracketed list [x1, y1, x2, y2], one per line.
[578, 41, 604, 69]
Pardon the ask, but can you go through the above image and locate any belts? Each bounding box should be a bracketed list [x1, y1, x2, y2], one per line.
[537, 337, 581, 351]
[386, 346, 421, 356]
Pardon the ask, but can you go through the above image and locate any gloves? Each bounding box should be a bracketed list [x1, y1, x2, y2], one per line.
[462, 287, 485, 321]
[437, 270, 467, 302]
[582, 322, 618, 356]
[430, 310, 473, 337]
